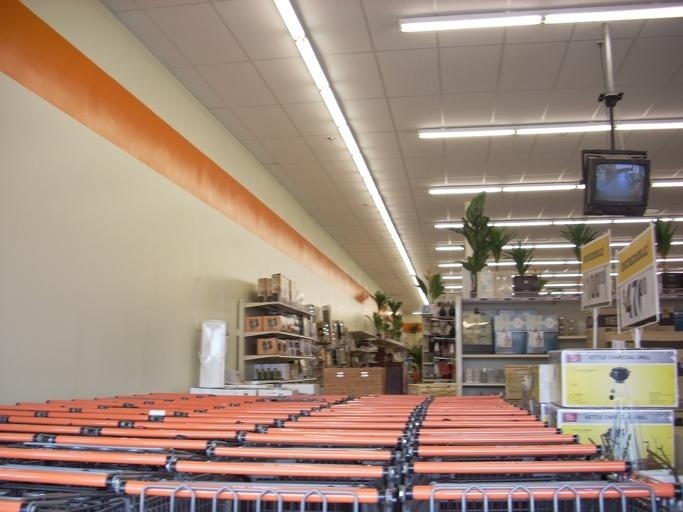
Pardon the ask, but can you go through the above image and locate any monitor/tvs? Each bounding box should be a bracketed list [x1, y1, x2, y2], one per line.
[583, 154, 650, 216]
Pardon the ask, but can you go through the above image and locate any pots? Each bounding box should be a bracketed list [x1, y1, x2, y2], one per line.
[610, 368, 630, 382]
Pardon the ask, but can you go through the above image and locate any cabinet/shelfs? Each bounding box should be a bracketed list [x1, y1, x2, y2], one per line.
[419, 302, 457, 395]
[236, 299, 318, 384]
[454, 296, 683, 397]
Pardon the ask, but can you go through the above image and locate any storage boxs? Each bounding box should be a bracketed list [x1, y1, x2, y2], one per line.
[257, 273, 298, 305]
[503, 345, 678, 470]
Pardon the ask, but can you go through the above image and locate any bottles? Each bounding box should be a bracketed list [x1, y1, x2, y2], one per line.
[439, 305, 455, 316]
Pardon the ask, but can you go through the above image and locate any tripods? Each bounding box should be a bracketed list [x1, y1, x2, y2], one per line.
[609, 368, 641, 470]
